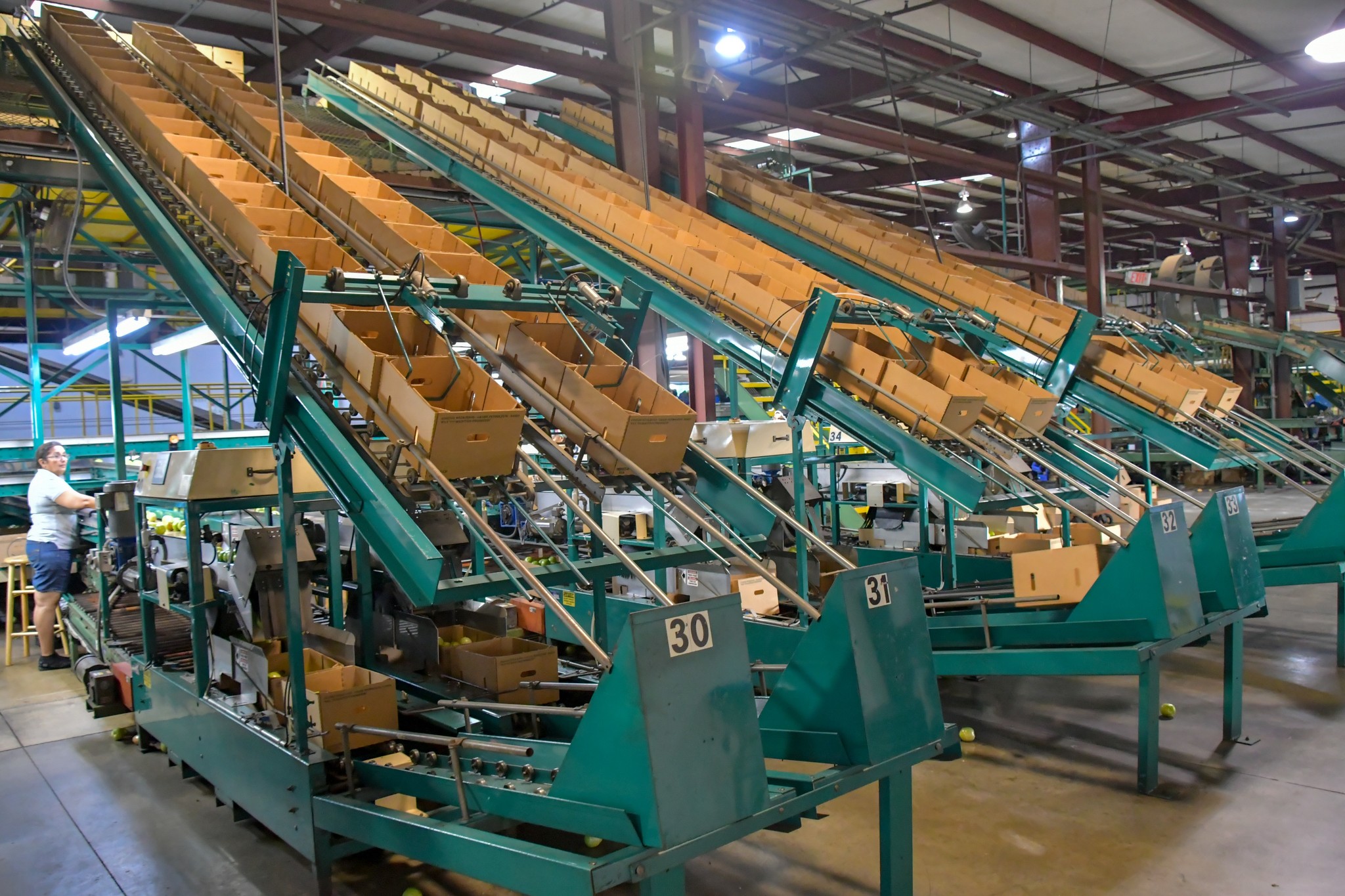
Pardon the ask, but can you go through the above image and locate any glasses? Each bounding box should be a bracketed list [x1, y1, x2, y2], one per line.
[43, 453, 70, 460]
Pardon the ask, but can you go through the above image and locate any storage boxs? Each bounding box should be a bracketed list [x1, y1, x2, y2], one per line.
[40, 1, 1288, 776]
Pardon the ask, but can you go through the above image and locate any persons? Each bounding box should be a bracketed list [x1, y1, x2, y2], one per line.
[23, 441, 100, 671]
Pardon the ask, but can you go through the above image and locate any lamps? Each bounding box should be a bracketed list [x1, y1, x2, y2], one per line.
[1302, 7, 1345, 65]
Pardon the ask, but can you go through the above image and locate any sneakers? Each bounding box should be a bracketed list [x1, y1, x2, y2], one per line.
[39, 652, 72, 671]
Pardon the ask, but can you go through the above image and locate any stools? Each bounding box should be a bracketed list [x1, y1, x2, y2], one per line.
[3, 554, 71, 666]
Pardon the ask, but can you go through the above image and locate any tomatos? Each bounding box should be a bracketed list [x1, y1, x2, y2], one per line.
[253, 507, 276, 512]
[268, 671, 281, 678]
[1160, 703, 1176, 717]
[438, 636, 472, 647]
[111, 726, 167, 754]
[95, 458, 103, 463]
[146, 507, 237, 563]
[524, 556, 557, 566]
[959, 727, 976, 742]
[989, 531, 996, 537]
[788, 547, 797, 553]
[565, 646, 576, 657]
